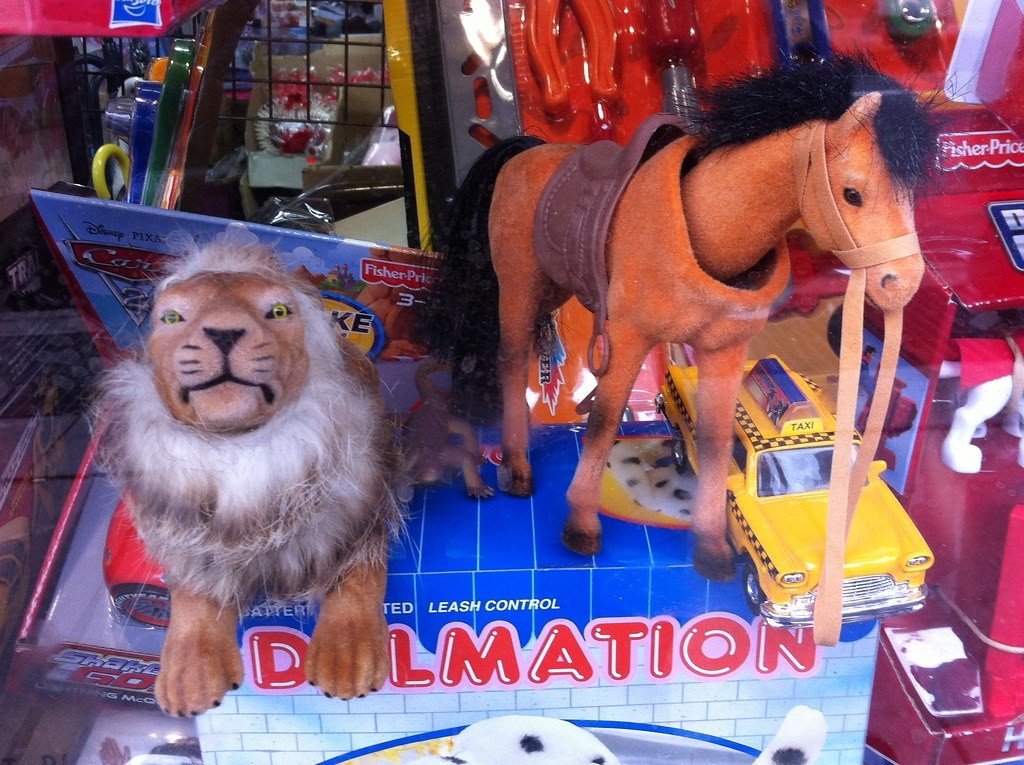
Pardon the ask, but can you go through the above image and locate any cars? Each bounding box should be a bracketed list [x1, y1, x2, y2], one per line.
[654, 354, 935, 629]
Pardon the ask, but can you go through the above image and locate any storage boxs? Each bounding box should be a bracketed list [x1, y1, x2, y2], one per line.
[301, 38, 405, 201]
[854, 111, 1024, 514]
[244, 51, 342, 191]
[197, 422, 880, 765]
[5, 403, 172, 716]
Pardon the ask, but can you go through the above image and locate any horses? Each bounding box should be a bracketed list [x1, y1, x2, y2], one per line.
[406, 51, 942, 582]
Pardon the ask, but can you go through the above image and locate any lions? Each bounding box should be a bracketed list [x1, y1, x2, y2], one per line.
[98, 234, 406, 719]
